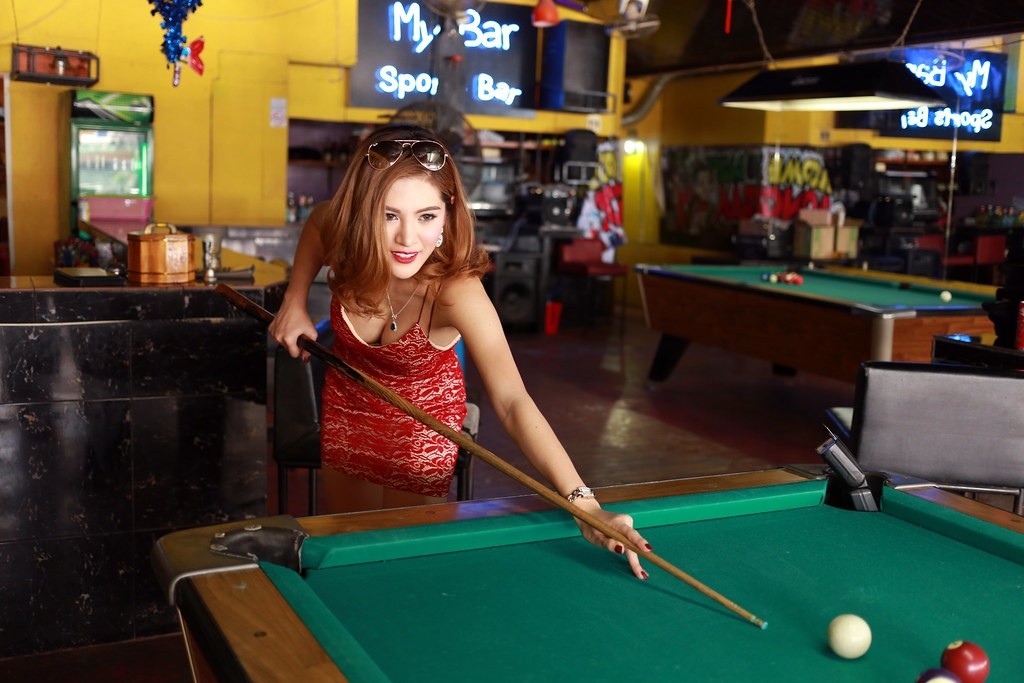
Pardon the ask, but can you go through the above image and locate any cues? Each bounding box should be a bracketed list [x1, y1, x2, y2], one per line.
[212, 279, 774, 634]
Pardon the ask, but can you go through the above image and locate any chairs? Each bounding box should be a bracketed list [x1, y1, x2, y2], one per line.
[824, 360, 1024, 517]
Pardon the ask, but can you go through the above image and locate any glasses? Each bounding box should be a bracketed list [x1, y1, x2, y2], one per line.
[364, 139, 449, 171]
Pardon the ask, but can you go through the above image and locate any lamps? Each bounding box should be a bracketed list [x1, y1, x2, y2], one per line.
[532, 0, 559, 27]
[717, 0, 947, 110]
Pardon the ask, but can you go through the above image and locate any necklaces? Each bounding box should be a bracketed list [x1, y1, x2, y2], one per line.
[386, 275, 422, 332]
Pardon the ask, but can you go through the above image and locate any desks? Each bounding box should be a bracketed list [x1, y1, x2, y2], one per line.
[151, 463, 1024, 683]
[634, 261, 1001, 381]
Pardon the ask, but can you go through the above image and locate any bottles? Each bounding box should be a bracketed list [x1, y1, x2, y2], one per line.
[54, 46, 66, 77]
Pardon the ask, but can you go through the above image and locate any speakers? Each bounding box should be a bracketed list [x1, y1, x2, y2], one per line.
[540, 18, 610, 114]
[841, 143, 872, 191]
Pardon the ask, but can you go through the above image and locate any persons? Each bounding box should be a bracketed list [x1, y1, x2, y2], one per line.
[669, 164, 733, 238]
[268, 125, 653, 580]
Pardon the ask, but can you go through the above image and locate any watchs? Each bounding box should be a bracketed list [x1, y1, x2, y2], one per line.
[567, 486, 595, 503]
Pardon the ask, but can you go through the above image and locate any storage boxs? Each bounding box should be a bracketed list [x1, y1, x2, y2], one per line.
[794, 209, 856, 260]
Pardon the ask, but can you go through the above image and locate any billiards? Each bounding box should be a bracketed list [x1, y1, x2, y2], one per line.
[916, 638, 991, 683]
[761, 270, 803, 285]
[940, 290, 953, 303]
[824, 612, 874, 661]
